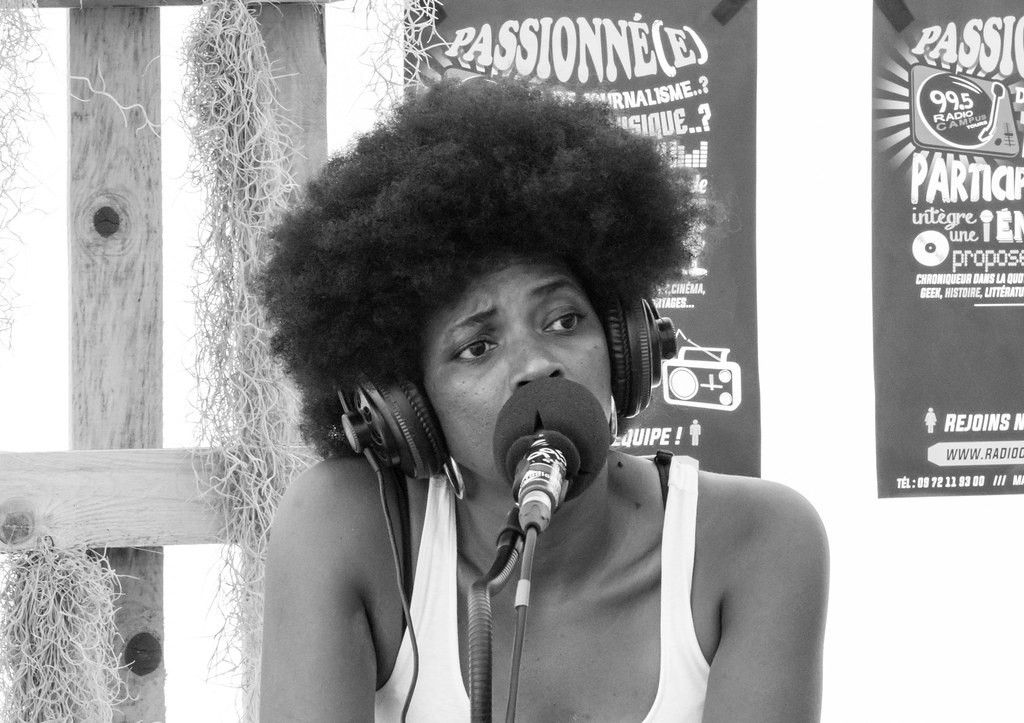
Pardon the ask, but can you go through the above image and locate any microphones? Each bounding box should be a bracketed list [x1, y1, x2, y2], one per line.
[493, 376, 613, 536]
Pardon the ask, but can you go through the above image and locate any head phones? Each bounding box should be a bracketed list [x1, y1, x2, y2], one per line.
[334, 293, 675, 499]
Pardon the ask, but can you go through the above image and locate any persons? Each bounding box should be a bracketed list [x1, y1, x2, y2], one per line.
[247, 76, 830, 723]
